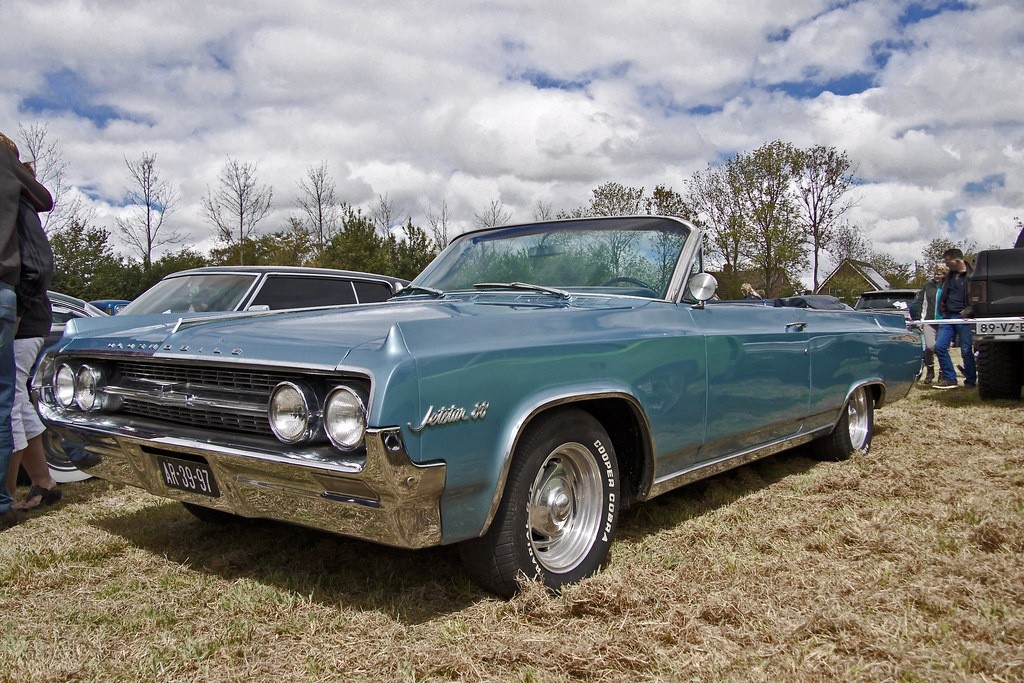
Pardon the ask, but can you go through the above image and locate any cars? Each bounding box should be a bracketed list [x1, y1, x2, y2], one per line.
[26, 216, 924, 597]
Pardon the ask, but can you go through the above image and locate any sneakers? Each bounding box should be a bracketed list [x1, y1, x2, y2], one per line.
[964, 380, 975, 387]
[932, 381, 958, 389]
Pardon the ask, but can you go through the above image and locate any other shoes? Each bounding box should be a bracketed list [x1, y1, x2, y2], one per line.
[924, 375, 935, 384]
[0, 507, 17, 531]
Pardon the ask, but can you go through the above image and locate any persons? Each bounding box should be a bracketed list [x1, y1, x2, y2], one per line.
[0, 132, 53, 533]
[932, 249, 974, 389]
[916, 263, 949, 384]
[741, 283, 762, 300]
[7, 161, 62, 512]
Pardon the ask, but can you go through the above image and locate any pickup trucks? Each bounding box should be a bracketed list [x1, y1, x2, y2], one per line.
[966, 228, 1024, 400]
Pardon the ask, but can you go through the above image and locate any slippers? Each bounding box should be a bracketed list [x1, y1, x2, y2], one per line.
[22, 485, 62, 511]
